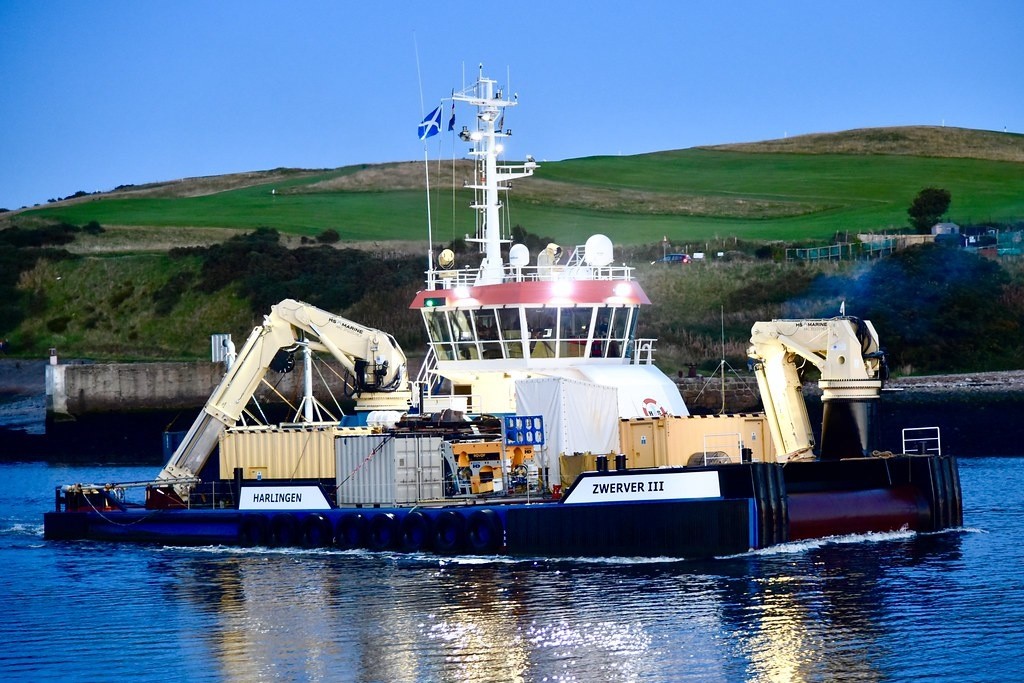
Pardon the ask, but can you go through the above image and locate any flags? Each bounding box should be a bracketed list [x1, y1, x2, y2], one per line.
[418, 103, 442, 140]
[448, 99, 455, 131]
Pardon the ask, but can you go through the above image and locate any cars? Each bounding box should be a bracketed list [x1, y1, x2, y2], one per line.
[650, 254, 693, 265]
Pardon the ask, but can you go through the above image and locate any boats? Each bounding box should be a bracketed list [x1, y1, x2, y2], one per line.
[38, 61, 969, 568]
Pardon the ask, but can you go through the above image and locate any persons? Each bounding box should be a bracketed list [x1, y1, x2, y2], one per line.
[104, 484, 118, 507]
[476, 316, 620, 358]
